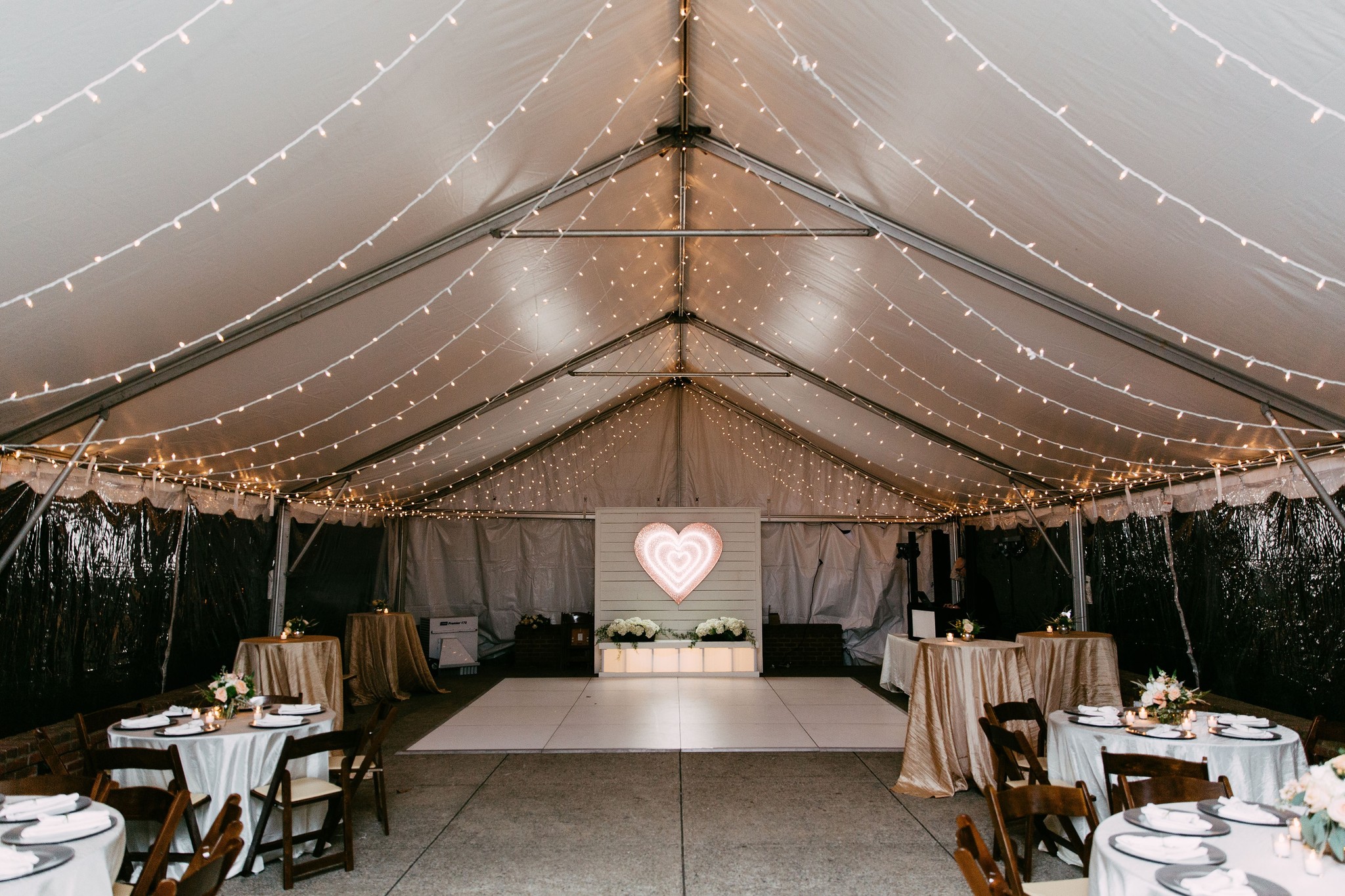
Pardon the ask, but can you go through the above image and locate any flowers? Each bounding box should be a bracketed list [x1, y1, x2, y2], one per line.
[953, 617, 980, 636]
[1131, 667, 1211, 712]
[1277, 752, 1345, 874]
[281, 613, 318, 634]
[595, 616, 755, 649]
[373, 599, 389, 609]
[519, 613, 551, 631]
[1040, 605, 1082, 631]
[197, 667, 258, 726]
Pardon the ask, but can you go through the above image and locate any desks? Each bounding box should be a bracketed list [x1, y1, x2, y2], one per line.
[107, 703, 339, 879]
[0, 799, 127, 896]
[235, 611, 450, 730]
[879, 632, 1123, 797]
[1043, 707, 1308, 870]
[1088, 795, 1345, 896]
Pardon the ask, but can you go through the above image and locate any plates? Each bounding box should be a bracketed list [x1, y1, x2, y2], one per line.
[1123, 806, 1231, 837]
[0, 794, 92, 824]
[269, 705, 326, 715]
[1125, 726, 1197, 739]
[112, 718, 179, 730]
[0, 814, 118, 843]
[1068, 715, 1133, 727]
[1154, 864, 1292, 896]
[247, 717, 311, 728]
[153, 722, 221, 736]
[1108, 831, 1226, 865]
[146, 707, 209, 717]
[1196, 798, 1302, 825]
[0, 844, 75, 882]
[1214, 715, 1277, 729]
[1063, 706, 1124, 716]
[1208, 726, 1282, 740]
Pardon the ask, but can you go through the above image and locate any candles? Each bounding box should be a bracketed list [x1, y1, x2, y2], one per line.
[281, 631, 287, 640]
[946, 633, 954, 642]
[384, 608, 388, 614]
[1047, 625, 1052, 633]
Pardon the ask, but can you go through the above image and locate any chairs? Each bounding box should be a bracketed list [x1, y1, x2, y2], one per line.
[952, 696, 1235, 896]
[25, 703, 387, 894]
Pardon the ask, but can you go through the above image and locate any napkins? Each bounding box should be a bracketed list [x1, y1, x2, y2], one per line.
[0, 794, 111, 878]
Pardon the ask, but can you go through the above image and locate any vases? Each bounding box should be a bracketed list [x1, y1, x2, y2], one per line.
[213, 698, 237, 720]
[611, 632, 656, 643]
[1155, 704, 1183, 726]
[700, 630, 743, 641]
[291, 629, 303, 638]
[960, 630, 974, 642]
[1056, 624, 1072, 634]
[374, 607, 382, 614]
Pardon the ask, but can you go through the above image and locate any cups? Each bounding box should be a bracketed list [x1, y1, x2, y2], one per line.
[946, 633, 954, 642]
[1046, 625, 1053, 633]
